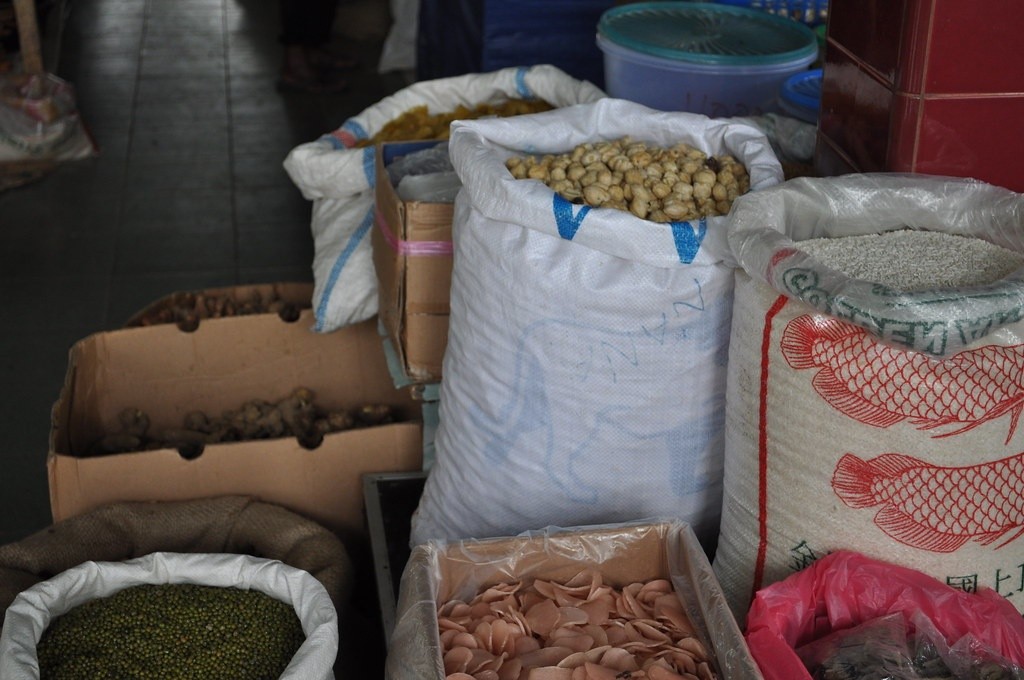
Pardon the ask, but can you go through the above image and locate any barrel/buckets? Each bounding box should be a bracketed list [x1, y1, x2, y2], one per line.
[594, 4, 820, 118]
